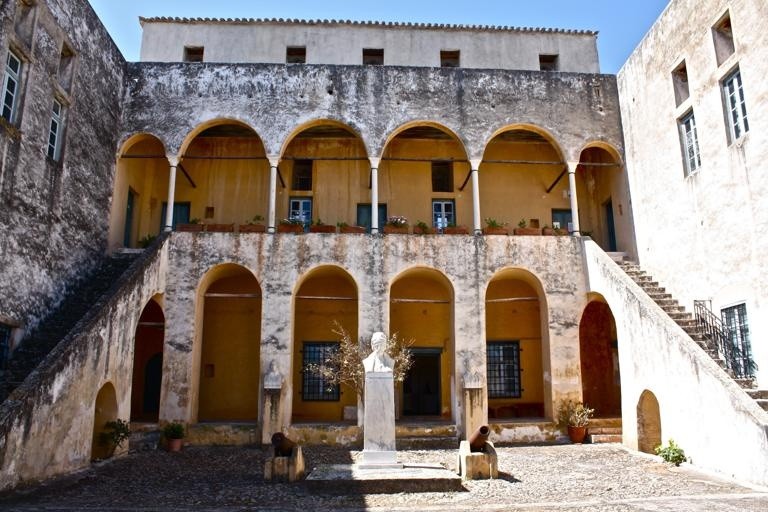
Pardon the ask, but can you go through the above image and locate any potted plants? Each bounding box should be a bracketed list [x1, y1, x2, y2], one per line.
[163, 421, 185, 451]
[559, 399, 595, 442]
[95, 431, 115, 458]
[174, 215, 570, 236]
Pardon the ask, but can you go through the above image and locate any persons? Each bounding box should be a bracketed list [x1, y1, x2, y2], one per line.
[462, 358, 484, 387]
[362, 332, 396, 373]
[264, 360, 285, 386]
